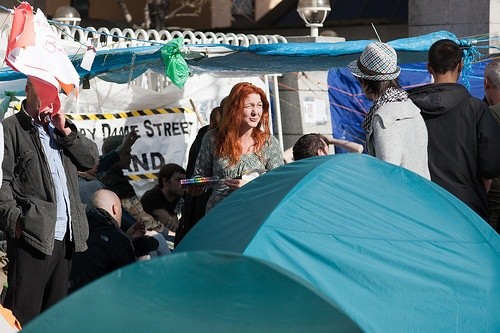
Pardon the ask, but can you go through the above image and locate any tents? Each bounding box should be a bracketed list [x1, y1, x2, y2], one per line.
[18, 154, 500, 332]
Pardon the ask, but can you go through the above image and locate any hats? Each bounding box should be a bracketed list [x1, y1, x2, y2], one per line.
[347, 42, 401, 81]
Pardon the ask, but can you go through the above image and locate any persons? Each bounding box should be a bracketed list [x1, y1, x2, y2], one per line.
[0, 70, 172, 333]
[173, 82, 283, 251]
[481, 58, 500, 234]
[293, 133, 365, 162]
[407, 39, 500, 224]
[347, 43, 432, 181]
[141, 163, 187, 251]
